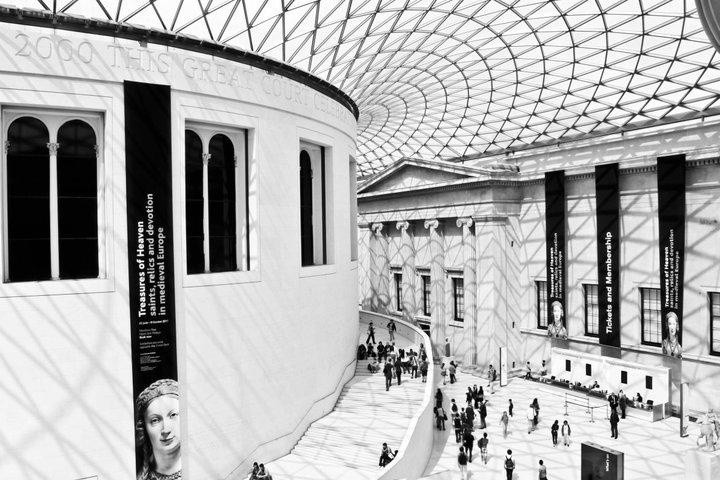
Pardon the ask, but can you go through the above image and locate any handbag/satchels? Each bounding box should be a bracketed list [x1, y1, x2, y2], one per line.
[444, 414, 447, 420]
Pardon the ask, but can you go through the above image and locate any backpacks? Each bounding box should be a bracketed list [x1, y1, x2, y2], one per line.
[454, 418, 461, 429]
[460, 453, 466, 464]
[506, 456, 513, 467]
[452, 402, 458, 412]
[478, 438, 483, 448]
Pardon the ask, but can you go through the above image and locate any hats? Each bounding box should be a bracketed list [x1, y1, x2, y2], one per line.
[665, 312, 679, 322]
[550, 301, 563, 309]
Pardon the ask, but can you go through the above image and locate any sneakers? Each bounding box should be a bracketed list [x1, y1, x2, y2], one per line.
[611, 435, 618, 439]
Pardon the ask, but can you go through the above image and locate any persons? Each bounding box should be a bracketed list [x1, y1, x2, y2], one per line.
[365, 322, 377, 344]
[502, 449, 516, 480]
[551, 419, 560, 448]
[661, 312, 682, 360]
[248, 461, 272, 479]
[631, 392, 642, 408]
[526, 398, 540, 434]
[523, 360, 532, 381]
[537, 359, 548, 383]
[377, 442, 398, 468]
[134, 379, 183, 479]
[561, 421, 571, 447]
[509, 398, 513, 417]
[608, 389, 627, 439]
[434, 361, 497, 480]
[386, 319, 397, 341]
[499, 411, 508, 437]
[589, 380, 601, 397]
[547, 301, 568, 341]
[357, 341, 428, 391]
[538, 460, 548, 480]
